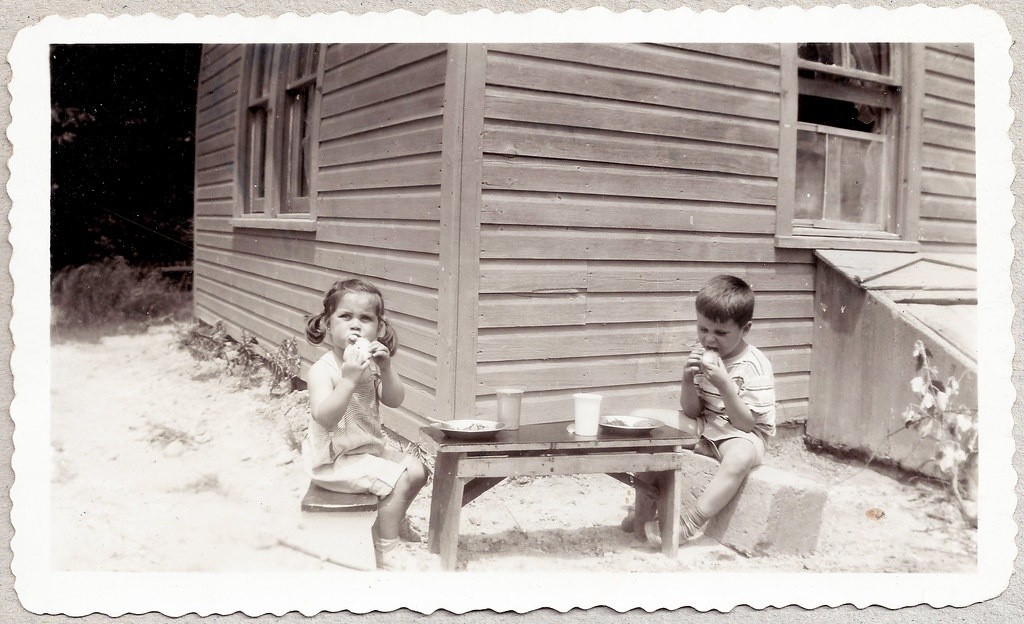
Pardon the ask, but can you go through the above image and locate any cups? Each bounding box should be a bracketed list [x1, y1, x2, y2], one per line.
[495, 388, 525, 431]
[572, 393, 604, 436]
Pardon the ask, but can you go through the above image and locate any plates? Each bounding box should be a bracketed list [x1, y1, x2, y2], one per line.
[430, 419, 508, 440]
[599, 416, 666, 434]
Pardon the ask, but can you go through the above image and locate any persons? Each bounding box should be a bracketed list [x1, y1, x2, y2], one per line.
[303, 278, 429, 572]
[620, 274, 777, 550]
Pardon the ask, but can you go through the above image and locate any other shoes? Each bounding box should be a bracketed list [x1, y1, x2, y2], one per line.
[399, 514, 422, 542]
[645, 521, 684, 548]
[622, 512, 635, 531]
[375, 539, 434, 574]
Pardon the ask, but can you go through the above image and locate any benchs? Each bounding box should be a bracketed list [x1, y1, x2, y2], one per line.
[417, 424, 700, 557]
[632, 410, 826, 563]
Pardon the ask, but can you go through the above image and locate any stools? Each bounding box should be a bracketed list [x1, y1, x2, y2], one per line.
[283, 485, 381, 573]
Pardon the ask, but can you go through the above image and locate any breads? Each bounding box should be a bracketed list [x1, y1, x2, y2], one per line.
[342, 334, 373, 362]
[702, 350, 720, 365]
